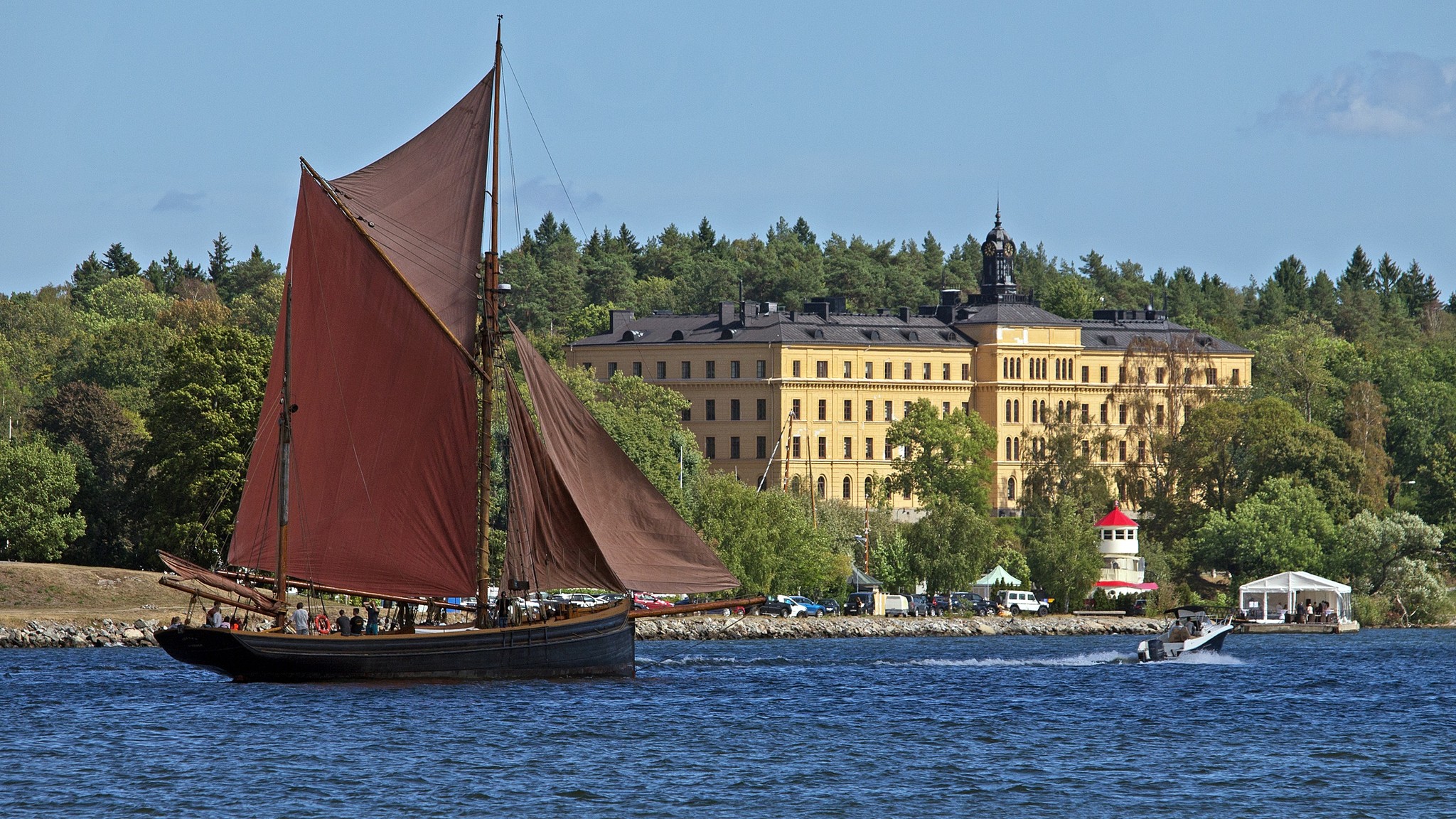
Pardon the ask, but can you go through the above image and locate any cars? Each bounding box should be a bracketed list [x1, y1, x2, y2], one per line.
[816, 598, 843, 616]
[459, 587, 676, 621]
[1133, 599, 1147, 616]
[674, 597, 730, 618]
[729, 593, 826, 617]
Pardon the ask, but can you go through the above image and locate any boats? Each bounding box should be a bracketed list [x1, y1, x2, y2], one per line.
[1136, 605, 1235, 663]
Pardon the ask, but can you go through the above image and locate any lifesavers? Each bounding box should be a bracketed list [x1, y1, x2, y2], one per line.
[314, 614, 331, 634]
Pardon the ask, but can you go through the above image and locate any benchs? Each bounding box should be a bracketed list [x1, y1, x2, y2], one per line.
[1073, 610, 1126, 617]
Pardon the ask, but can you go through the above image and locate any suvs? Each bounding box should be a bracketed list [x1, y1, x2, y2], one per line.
[998, 590, 1049, 617]
[883, 591, 1000, 618]
[842, 591, 876, 617]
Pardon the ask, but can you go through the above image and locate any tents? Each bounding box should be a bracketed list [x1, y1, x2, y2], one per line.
[1238, 569, 1352, 624]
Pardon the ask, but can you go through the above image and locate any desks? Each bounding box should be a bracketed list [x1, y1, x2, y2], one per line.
[1267, 609, 1335, 623]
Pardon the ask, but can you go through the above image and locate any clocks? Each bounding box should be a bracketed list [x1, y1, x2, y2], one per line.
[1003, 241, 1014, 257]
[983, 241, 997, 258]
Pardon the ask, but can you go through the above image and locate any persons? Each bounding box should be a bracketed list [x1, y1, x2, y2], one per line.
[1169, 616, 1197, 641]
[1276, 600, 1328, 625]
[169, 590, 513, 634]
[855, 597, 863, 618]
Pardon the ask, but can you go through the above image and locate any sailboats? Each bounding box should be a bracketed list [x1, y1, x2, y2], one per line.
[148, 12, 772, 686]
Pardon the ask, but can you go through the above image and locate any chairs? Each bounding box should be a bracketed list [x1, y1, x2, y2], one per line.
[1168, 625, 1181, 643]
[1180, 626, 1197, 642]
